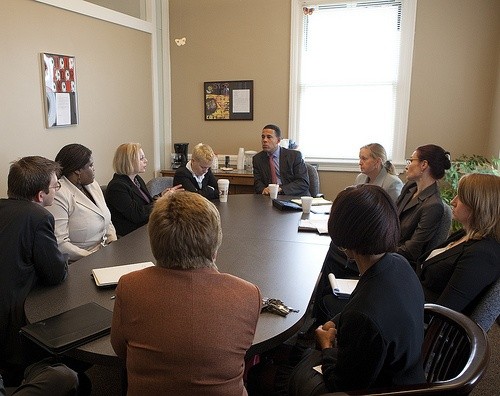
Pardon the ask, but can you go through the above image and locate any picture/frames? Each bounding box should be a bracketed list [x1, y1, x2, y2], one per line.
[40, 52, 80, 129]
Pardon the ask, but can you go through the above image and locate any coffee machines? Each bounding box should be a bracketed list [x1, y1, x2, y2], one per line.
[172, 143, 189, 169]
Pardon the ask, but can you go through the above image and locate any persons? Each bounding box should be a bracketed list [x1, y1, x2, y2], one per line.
[172, 142, 220, 200]
[44, 143, 117, 265]
[314, 145, 450, 299]
[0, 156, 95, 388]
[0, 357, 80, 396]
[296, 173, 500, 348]
[252, 124, 311, 197]
[105, 143, 185, 238]
[110, 191, 263, 396]
[286, 184, 426, 396]
[354, 141, 403, 202]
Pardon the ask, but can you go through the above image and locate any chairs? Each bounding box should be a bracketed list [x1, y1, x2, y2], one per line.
[102, 165, 500, 396]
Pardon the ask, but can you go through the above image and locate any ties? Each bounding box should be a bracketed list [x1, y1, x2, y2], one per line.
[269, 154, 278, 184]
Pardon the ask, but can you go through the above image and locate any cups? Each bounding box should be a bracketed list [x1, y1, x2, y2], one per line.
[216, 179, 230, 202]
[225, 156, 230, 168]
[269, 184, 279, 199]
[237, 148, 245, 170]
[301, 196, 313, 214]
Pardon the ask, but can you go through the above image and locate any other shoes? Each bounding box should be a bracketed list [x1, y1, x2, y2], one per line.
[297, 322, 324, 340]
[23, 353, 94, 377]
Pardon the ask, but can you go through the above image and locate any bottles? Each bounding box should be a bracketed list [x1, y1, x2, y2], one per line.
[211, 156, 218, 170]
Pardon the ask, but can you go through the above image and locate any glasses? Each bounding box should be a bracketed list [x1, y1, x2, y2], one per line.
[49, 181, 62, 191]
[139, 154, 148, 161]
[406, 156, 431, 167]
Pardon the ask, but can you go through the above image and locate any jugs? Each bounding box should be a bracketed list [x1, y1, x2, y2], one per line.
[171, 153, 184, 163]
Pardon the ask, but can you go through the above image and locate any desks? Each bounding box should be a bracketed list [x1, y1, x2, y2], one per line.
[160, 168, 255, 185]
[23, 191, 335, 396]
